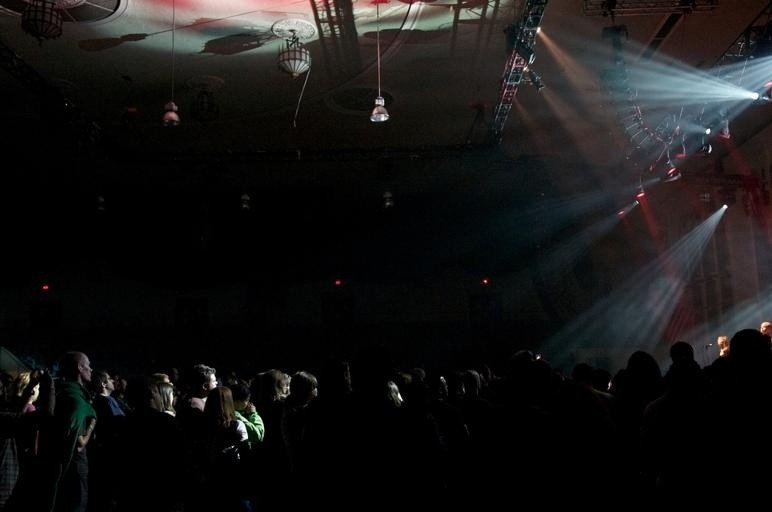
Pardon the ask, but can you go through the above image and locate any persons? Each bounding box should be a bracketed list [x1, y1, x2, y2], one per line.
[0, 320, 772, 511]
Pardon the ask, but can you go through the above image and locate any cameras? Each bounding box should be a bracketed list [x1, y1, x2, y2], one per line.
[37, 367, 47, 376]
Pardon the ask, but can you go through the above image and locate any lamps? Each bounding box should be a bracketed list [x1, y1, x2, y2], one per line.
[21, 0, 64, 48]
[369, 3, 390, 123]
[272, 17, 318, 79]
